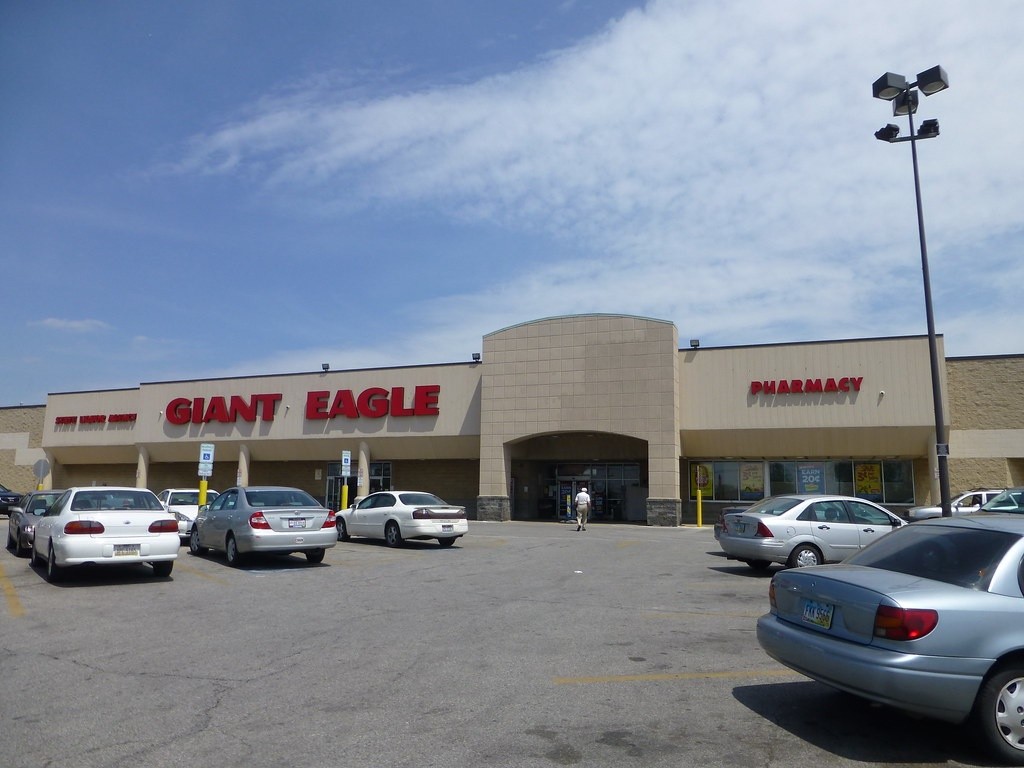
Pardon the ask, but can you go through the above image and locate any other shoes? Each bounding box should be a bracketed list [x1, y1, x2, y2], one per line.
[577, 525, 581, 531]
[582, 528, 586, 530]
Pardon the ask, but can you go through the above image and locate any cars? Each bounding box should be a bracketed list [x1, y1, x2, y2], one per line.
[0, 484, 225, 580]
[189, 486, 337, 563]
[714, 488, 1024, 768]
[335, 491, 468, 548]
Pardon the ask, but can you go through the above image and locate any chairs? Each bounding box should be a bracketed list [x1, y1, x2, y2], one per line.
[31, 499, 47, 512]
[75, 499, 92, 509]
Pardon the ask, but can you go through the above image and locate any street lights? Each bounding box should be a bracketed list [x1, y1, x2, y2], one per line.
[872, 65, 952, 517]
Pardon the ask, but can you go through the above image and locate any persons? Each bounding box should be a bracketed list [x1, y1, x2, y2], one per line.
[574, 488, 591, 531]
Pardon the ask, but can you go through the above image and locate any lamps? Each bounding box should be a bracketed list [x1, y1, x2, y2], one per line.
[472, 353, 481, 362]
[322, 364, 330, 371]
[690, 340, 699, 348]
